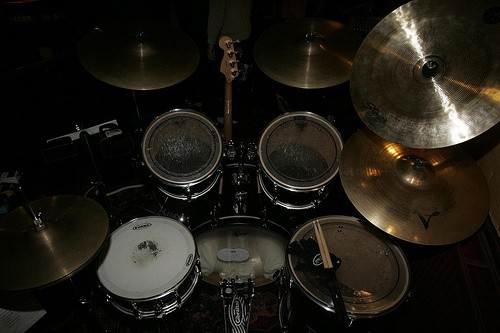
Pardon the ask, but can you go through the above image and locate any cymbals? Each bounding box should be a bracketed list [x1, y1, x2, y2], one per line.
[339, 127, 491, 246]
[350, 0, 500, 149]
[76, 22, 200, 91]
[0, 194, 109, 290]
[253, 16, 362, 90]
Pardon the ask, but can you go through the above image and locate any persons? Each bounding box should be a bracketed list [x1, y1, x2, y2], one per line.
[282, 0, 304, 18]
[207, 0, 252, 61]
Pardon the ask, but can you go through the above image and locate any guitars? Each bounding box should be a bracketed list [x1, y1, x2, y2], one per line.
[218, 35, 259, 166]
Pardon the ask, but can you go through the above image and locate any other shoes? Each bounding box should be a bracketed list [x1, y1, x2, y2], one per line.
[276, 93, 288, 112]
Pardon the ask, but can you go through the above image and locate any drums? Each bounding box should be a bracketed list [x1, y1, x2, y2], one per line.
[96, 215, 201, 319]
[187, 162, 294, 290]
[141, 110, 222, 201]
[259, 111, 344, 210]
[279, 214, 411, 333]
